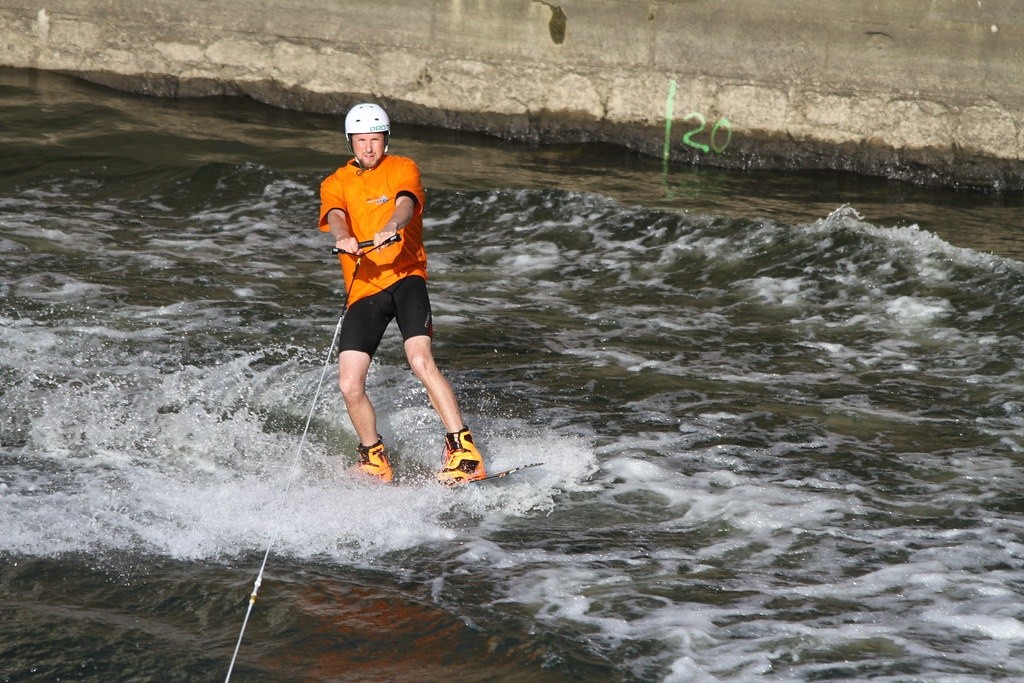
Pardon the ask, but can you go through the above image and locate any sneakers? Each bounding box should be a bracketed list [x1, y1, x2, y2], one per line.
[354, 435, 391, 482]
[435, 426, 485, 484]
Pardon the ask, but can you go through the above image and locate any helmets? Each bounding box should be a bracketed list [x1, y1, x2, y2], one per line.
[344, 103, 390, 142]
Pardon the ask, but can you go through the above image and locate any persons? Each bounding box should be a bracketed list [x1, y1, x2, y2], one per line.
[318, 102, 488, 485]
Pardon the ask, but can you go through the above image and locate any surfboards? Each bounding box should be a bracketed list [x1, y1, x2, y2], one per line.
[374, 460, 546, 488]
